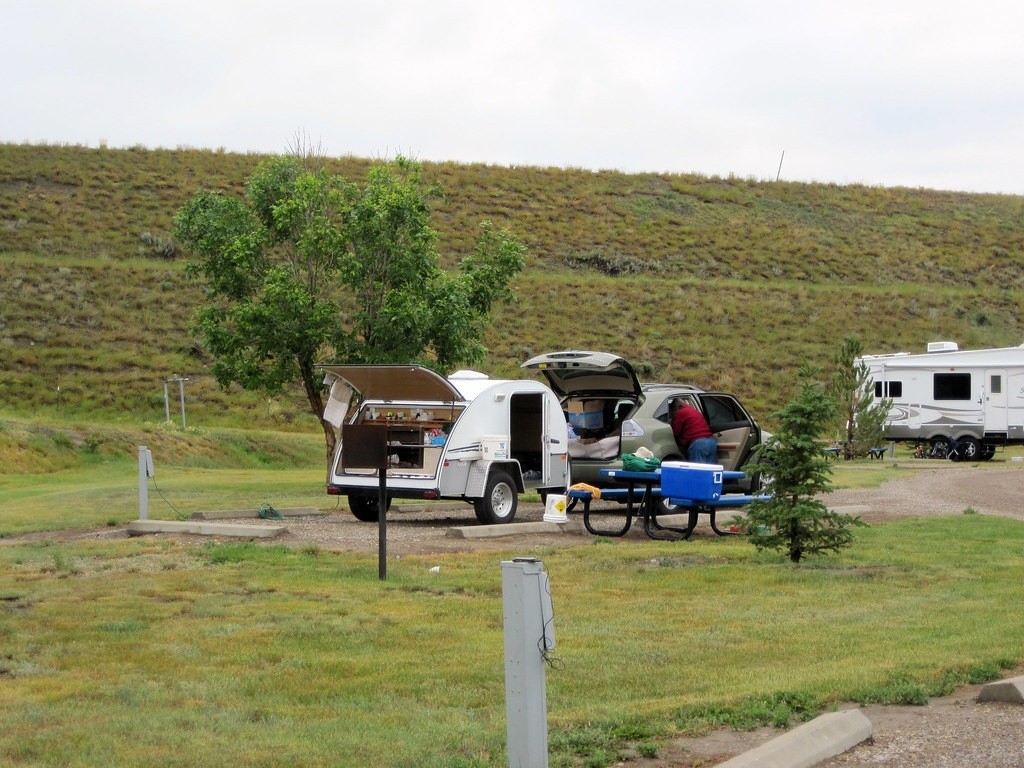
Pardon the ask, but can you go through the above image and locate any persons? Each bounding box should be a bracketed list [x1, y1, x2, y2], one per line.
[672, 398, 716, 464]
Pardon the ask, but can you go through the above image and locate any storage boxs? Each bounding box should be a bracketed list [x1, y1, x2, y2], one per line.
[660, 460, 724, 501]
[562, 399, 605, 430]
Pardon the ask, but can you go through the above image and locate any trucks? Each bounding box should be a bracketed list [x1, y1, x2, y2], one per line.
[846, 340, 1024, 463]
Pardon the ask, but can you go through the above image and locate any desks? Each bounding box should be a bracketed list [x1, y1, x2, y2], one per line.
[598, 468, 747, 540]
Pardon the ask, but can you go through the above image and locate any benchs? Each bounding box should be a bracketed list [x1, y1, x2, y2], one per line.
[823, 448, 841, 461]
[570, 488, 663, 537]
[870, 448, 888, 459]
[668, 495, 772, 540]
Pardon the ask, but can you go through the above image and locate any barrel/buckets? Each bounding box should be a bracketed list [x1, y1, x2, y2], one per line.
[479, 435, 508, 460]
[543, 495, 569, 524]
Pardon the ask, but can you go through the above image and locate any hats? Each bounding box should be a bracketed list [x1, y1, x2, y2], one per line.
[632, 447, 654, 459]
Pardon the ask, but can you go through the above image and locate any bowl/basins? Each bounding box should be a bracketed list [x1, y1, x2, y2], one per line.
[1010, 457, 1023, 462]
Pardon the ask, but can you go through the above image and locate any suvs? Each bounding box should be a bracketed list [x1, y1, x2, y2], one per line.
[518, 351, 782, 497]
